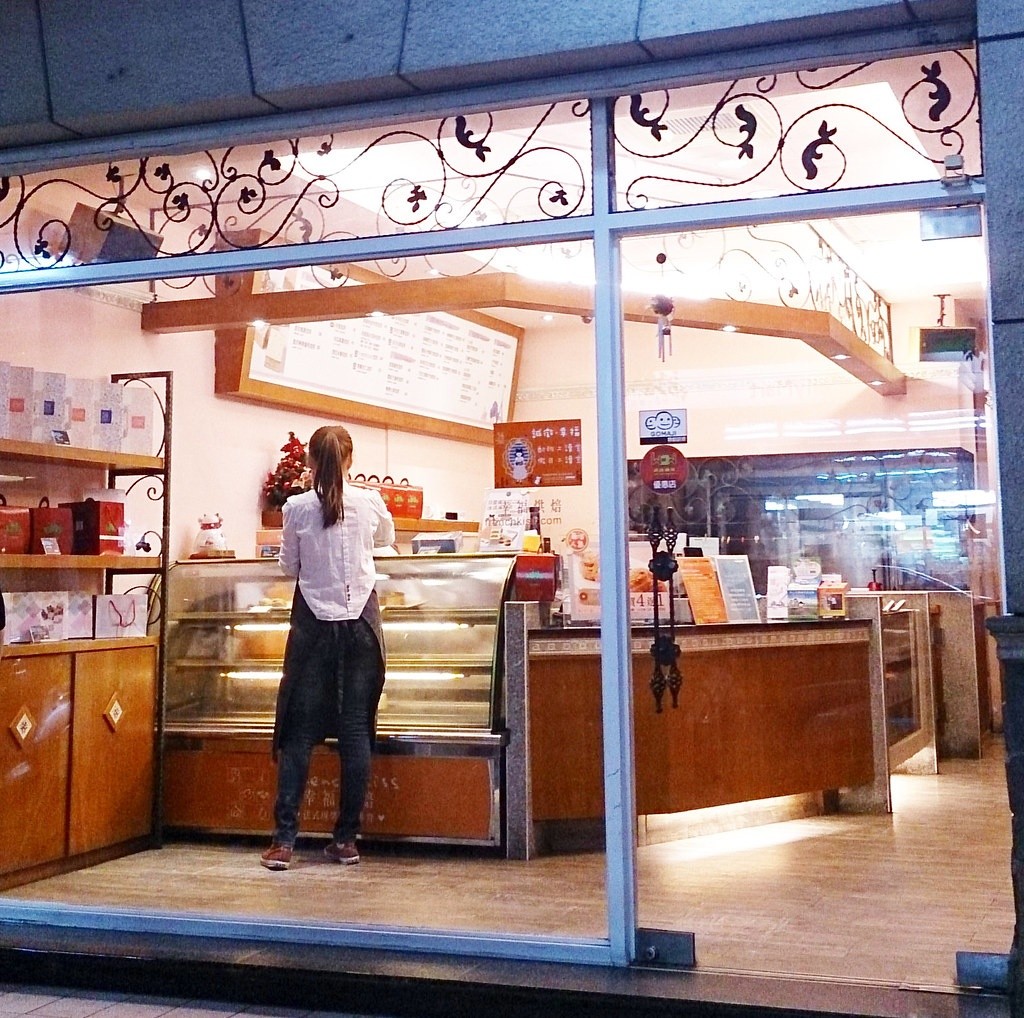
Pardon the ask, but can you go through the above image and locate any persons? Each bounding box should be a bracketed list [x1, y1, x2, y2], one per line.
[259, 423, 396, 870]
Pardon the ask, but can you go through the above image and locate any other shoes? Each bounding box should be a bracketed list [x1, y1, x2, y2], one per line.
[325, 836, 360, 864]
[260, 842, 293, 868]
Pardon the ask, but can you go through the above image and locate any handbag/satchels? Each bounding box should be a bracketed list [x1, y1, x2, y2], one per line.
[92, 594, 148, 636]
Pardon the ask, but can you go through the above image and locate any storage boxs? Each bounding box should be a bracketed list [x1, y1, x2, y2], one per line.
[345, 467, 425, 520]
[3, 487, 152, 644]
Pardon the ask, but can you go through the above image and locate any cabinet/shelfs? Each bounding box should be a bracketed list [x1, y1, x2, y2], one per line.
[150, 540, 519, 856]
[875, 605, 924, 741]
[0, 438, 172, 891]
